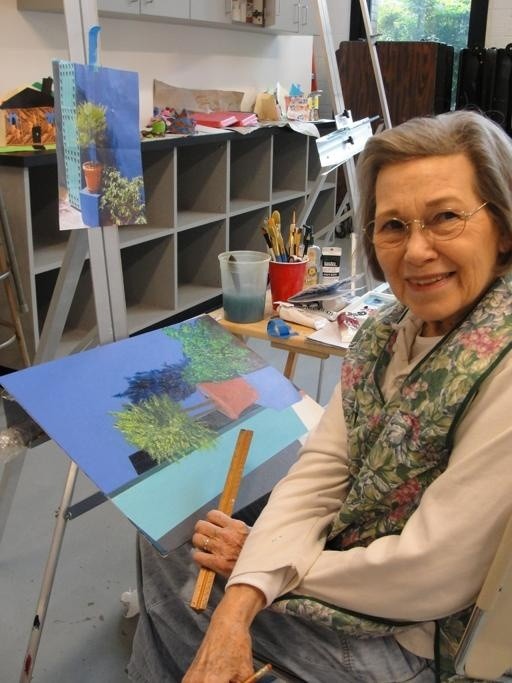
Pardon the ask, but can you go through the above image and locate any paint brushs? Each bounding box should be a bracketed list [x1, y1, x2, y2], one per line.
[261, 211, 303, 264]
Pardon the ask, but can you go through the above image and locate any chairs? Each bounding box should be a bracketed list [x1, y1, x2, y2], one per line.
[433, 511, 512, 682]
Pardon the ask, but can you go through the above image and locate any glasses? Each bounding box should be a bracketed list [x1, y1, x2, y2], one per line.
[361, 201, 489, 250]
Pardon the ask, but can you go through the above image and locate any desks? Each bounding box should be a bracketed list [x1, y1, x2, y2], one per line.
[198, 273, 401, 400]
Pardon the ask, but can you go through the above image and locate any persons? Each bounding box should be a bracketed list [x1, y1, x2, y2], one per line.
[120, 107, 512, 682]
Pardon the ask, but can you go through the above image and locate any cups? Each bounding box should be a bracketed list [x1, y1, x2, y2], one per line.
[272, 255, 309, 313]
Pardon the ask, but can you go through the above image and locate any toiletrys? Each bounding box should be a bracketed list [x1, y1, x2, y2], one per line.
[298, 224, 322, 290]
[320, 247, 342, 284]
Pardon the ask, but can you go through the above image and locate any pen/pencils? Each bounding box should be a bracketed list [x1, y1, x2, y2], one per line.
[245, 664, 272, 683]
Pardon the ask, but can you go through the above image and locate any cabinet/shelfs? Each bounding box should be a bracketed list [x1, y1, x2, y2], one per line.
[0, 113, 343, 374]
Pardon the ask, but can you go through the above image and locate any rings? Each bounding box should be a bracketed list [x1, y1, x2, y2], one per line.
[203, 537, 211, 551]
